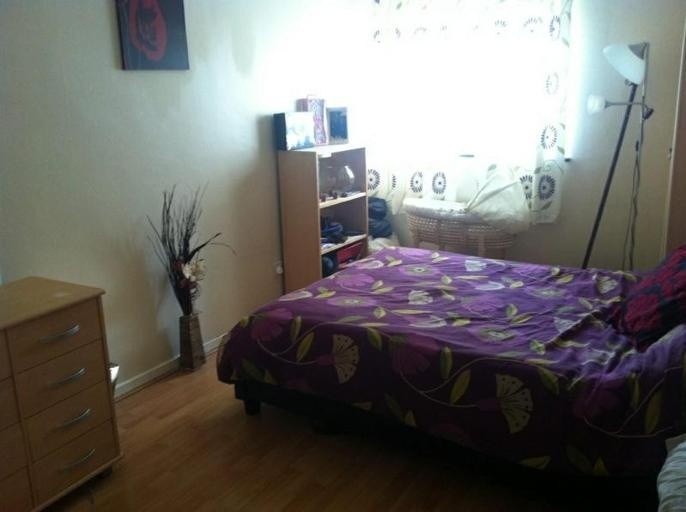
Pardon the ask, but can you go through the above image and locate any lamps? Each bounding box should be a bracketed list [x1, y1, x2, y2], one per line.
[586, 94, 656, 275]
[581, 40, 649, 271]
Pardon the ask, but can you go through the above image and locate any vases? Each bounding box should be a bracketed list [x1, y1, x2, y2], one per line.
[179, 315, 206, 368]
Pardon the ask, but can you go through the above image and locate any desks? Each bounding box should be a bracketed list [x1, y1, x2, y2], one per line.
[403, 198, 513, 260]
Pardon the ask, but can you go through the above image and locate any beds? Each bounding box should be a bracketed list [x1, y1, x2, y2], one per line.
[235, 245, 686, 490]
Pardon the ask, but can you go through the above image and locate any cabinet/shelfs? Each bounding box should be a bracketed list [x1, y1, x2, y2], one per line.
[0, 275, 126, 507]
[277, 139, 370, 294]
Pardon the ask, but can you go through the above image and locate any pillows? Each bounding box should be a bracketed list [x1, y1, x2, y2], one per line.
[611, 247, 686, 352]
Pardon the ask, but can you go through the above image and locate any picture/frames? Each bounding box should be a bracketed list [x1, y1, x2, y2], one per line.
[116, 1, 192, 71]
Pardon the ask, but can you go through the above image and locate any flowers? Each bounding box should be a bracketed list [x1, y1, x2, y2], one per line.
[145, 182, 217, 318]
